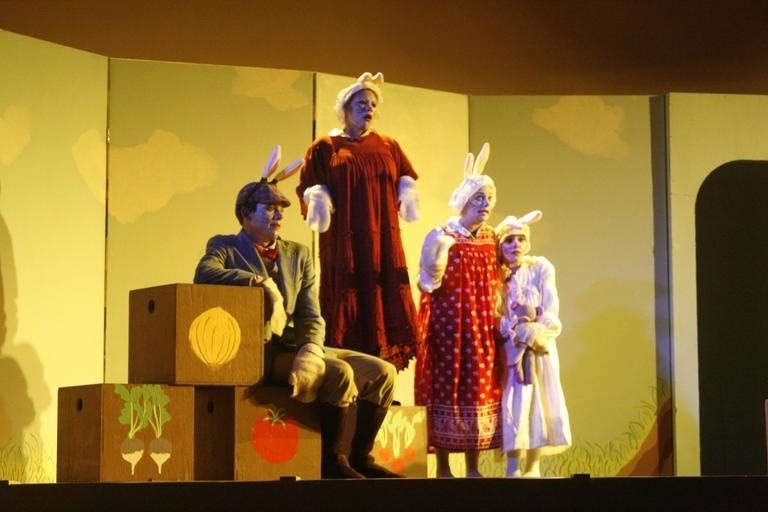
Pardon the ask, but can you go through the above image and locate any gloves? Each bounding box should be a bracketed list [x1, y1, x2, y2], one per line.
[515, 321, 549, 355]
[262, 278, 288, 338]
[289, 343, 325, 403]
[398, 175, 419, 222]
[419, 235, 456, 293]
[303, 184, 336, 233]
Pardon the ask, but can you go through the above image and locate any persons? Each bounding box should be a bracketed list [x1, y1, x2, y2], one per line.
[195, 142, 404, 479]
[495, 211, 572, 477]
[295, 73, 423, 372]
[417, 142, 502, 477]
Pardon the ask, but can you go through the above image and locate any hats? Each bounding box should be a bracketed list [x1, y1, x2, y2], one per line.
[449, 142, 497, 214]
[335, 72, 383, 121]
[495, 210, 542, 246]
[236, 145, 305, 206]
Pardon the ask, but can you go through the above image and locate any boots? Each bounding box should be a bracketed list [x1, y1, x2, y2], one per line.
[349, 397, 406, 478]
[320, 400, 368, 479]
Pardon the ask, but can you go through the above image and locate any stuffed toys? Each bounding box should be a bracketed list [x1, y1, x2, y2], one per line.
[509, 300, 544, 385]
